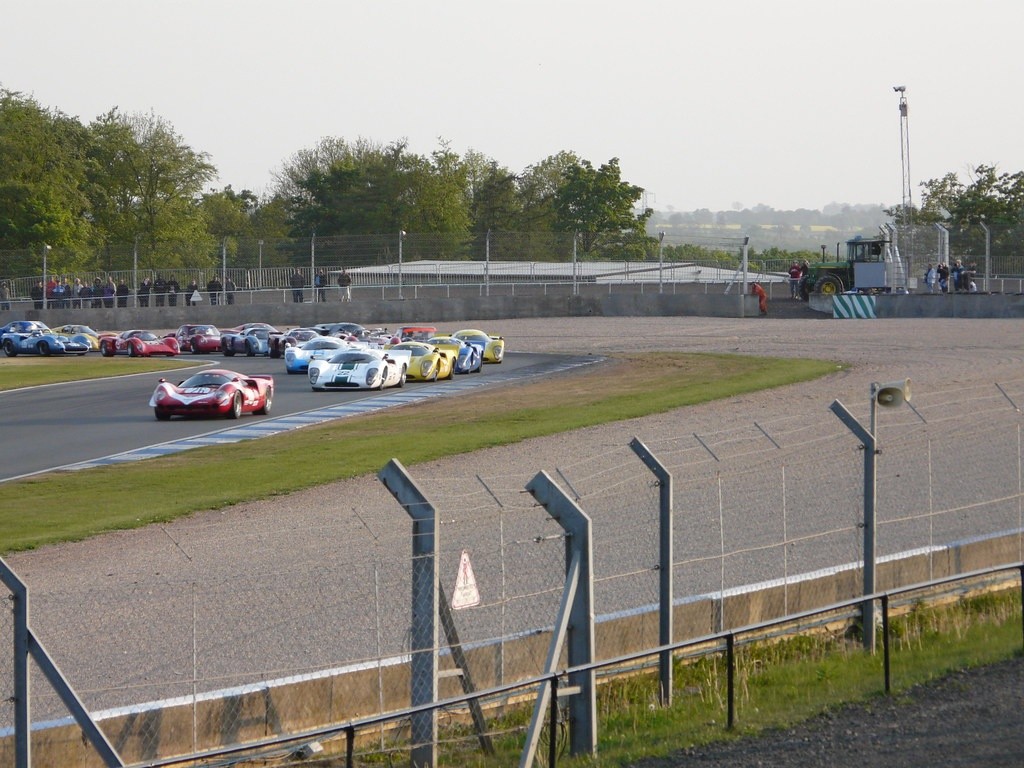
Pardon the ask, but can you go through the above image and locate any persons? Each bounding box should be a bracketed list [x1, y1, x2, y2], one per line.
[925, 263, 935, 293]
[30, 275, 128, 309]
[337, 269, 352, 301]
[206, 275, 237, 305]
[314, 270, 327, 301]
[137, 274, 180, 306]
[937, 262, 948, 292]
[185, 280, 198, 306]
[0, 282, 10, 310]
[951, 260, 976, 291]
[788, 259, 809, 298]
[750, 282, 768, 315]
[290, 268, 306, 302]
[857, 247, 865, 258]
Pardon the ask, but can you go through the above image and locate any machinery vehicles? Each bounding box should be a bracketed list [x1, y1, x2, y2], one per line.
[797, 236, 903, 301]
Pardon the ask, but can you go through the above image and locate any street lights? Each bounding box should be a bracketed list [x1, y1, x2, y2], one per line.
[42, 245, 53, 309]
[222, 236, 230, 306]
[572, 228, 583, 295]
[934, 222, 950, 294]
[743, 235, 751, 294]
[978, 220, 991, 292]
[310, 232, 318, 302]
[398, 230, 407, 301]
[485, 229, 493, 297]
[879, 222, 898, 293]
[892, 84, 917, 292]
[658, 230, 666, 295]
[132, 236, 140, 306]
[257, 239, 265, 292]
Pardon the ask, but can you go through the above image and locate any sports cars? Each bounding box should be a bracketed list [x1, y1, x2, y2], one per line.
[97, 329, 181, 357]
[147, 369, 278, 417]
[163, 322, 441, 373]
[0, 321, 50, 350]
[387, 341, 460, 384]
[429, 335, 486, 375]
[0, 328, 92, 357]
[305, 348, 412, 392]
[435, 329, 505, 365]
[47, 324, 118, 352]
